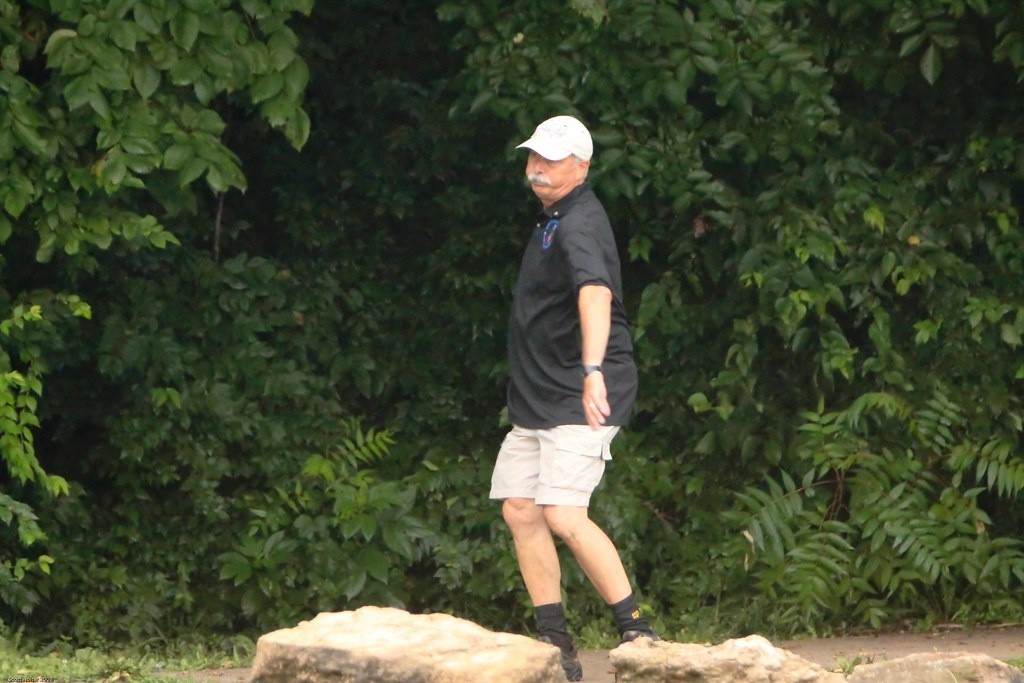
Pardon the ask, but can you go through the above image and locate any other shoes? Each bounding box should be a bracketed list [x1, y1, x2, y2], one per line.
[620, 629, 662, 642]
[538, 636, 582, 681]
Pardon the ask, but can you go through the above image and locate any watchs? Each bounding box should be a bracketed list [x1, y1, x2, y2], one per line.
[582, 365, 603, 376]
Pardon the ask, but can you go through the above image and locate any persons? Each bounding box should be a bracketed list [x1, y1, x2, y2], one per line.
[490, 115, 659, 681]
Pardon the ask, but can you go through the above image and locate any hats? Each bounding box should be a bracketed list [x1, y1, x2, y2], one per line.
[515, 116, 593, 161]
